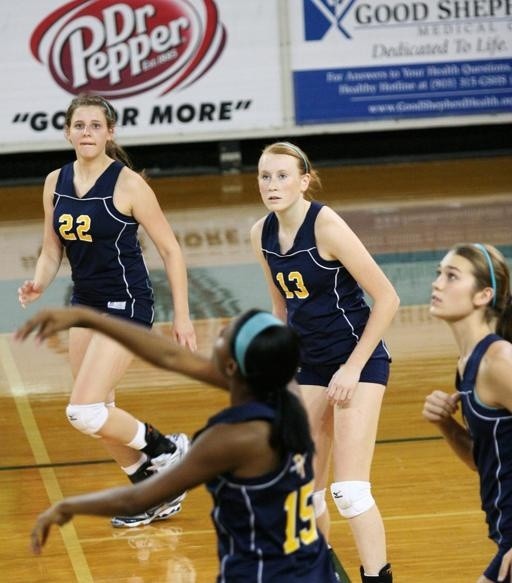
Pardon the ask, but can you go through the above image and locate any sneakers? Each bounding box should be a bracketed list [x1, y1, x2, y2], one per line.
[110, 498, 185, 528]
[147, 430, 193, 510]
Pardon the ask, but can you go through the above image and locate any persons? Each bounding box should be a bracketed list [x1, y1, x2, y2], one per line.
[248, 141, 400, 583]
[18, 95, 197, 527]
[14, 307, 341, 582]
[422, 242, 512, 583]
